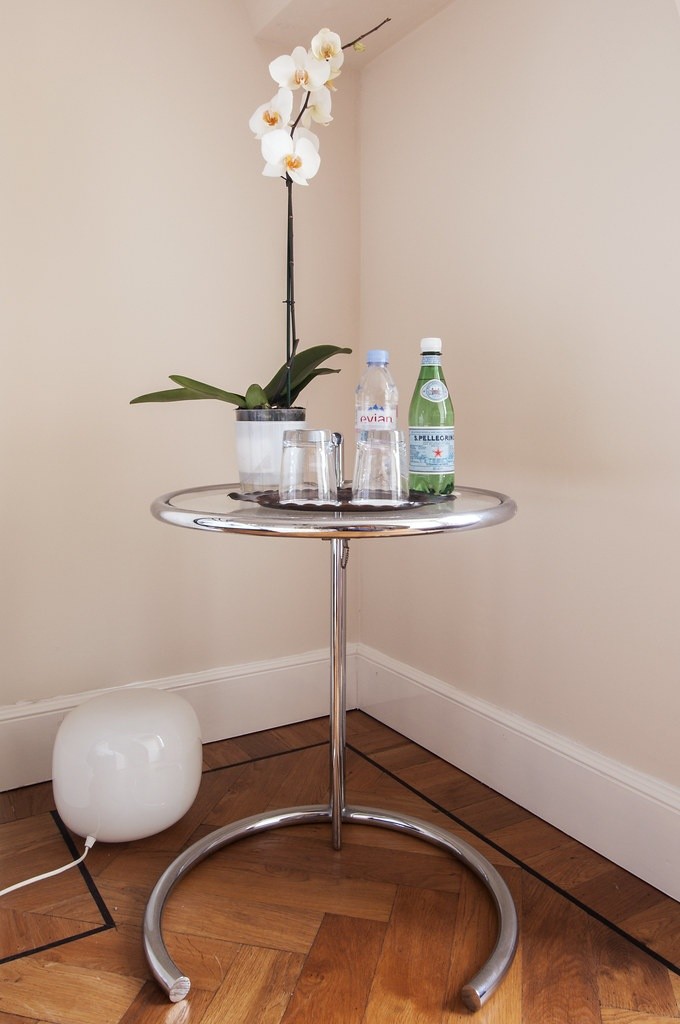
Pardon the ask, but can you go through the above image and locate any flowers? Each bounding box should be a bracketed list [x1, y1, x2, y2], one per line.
[128, 25, 353, 409]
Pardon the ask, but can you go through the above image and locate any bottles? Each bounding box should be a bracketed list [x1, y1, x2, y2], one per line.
[409, 338, 455, 495]
[352, 350, 398, 431]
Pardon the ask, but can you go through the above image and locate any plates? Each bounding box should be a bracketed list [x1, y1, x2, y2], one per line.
[227, 488, 458, 513]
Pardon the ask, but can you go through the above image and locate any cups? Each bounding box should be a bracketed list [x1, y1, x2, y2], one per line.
[330, 433, 345, 490]
[350, 430, 409, 503]
[279, 429, 337, 505]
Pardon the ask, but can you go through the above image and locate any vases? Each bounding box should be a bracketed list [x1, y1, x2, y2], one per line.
[237, 405, 307, 495]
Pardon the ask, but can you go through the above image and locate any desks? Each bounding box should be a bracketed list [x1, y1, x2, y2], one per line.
[140, 484, 518, 1011]
